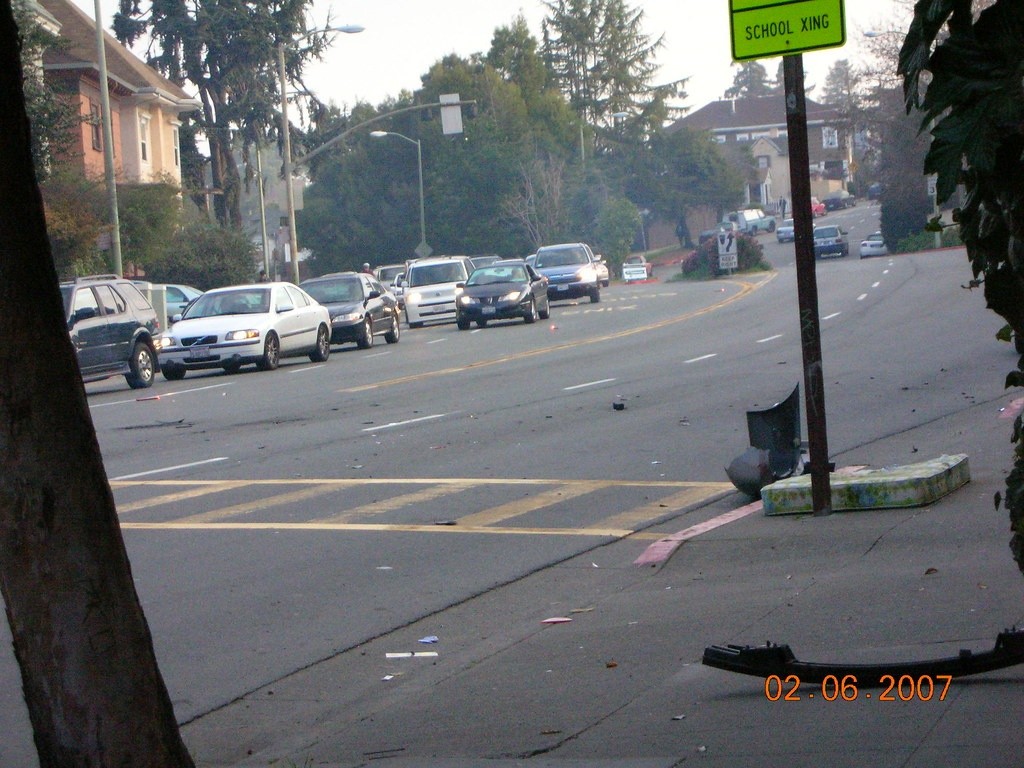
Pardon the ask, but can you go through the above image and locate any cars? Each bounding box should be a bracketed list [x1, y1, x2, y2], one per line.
[810, 196, 828, 220]
[594, 260, 610, 289]
[160, 284, 222, 319]
[859, 234, 889, 260]
[820, 189, 857, 210]
[867, 183, 884, 200]
[373, 253, 536, 329]
[775, 218, 817, 243]
[294, 271, 402, 350]
[154, 281, 333, 381]
[624, 254, 654, 278]
[455, 261, 552, 331]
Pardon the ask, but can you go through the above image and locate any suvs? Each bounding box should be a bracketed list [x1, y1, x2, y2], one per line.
[812, 224, 850, 258]
[56, 274, 161, 390]
[722, 209, 777, 237]
[532, 243, 602, 305]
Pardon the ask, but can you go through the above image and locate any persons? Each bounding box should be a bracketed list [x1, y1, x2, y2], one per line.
[510, 267, 531, 283]
[779, 196, 786, 219]
[362, 262, 375, 276]
[259, 270, 272, 282]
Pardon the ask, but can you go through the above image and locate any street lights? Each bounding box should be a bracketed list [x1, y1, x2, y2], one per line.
[277, 24, 367, 286]
[580, 112, 630, 176]
[369, 130, 427, 261]
